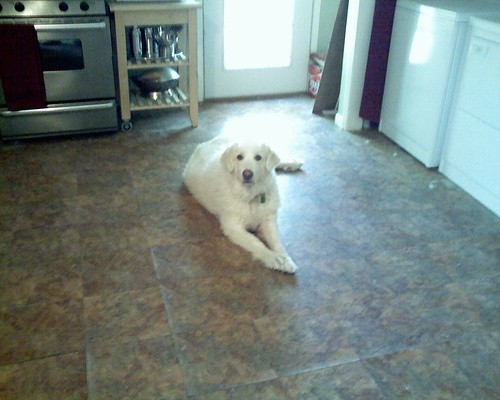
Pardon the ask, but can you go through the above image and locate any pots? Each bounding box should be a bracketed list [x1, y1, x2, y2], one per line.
[130, 68, 180, 92]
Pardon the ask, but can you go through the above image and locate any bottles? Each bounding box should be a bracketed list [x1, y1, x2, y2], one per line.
[153, 25, 179, 58]
[144, 27, 154, 60]
[133, 25, 143, 56]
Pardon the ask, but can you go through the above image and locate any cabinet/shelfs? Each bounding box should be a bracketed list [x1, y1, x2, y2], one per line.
[437, 19, 499, 217]
[377, 1, 467, 168]
[108, 0, 202, 128]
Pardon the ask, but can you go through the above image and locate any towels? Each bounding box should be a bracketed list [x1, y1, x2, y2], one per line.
[0, 23, 47, 111]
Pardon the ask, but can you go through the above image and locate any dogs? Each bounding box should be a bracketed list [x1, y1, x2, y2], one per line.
[182, 129, 299, 275]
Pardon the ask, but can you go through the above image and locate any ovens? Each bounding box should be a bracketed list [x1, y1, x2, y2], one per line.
[0, 16, 118, 141]
